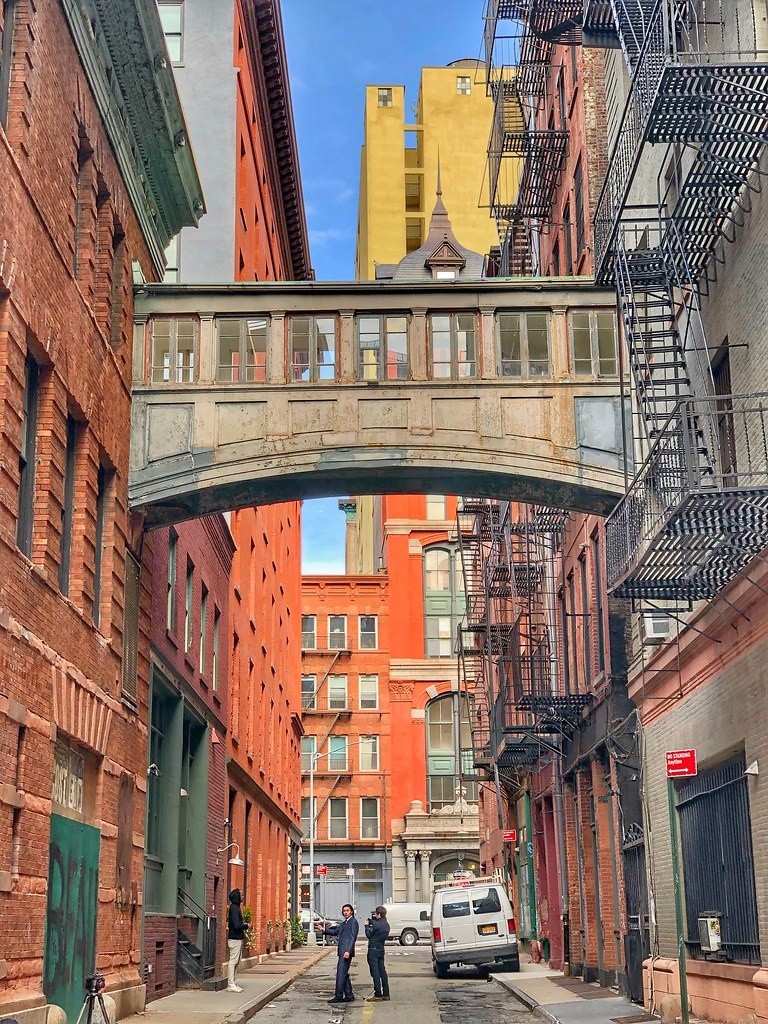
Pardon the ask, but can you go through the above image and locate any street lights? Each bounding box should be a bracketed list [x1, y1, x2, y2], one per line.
[307, 739, 377, 945]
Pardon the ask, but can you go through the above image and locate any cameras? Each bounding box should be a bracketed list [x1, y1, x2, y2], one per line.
[86, 975, 106, 993]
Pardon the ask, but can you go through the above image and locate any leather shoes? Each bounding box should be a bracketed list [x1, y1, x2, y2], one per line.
[367, 996, 382, 1002]
[327, 998, 343, 1003]
[344, 997, 354, 1002]
[382, 995, 390, 1000]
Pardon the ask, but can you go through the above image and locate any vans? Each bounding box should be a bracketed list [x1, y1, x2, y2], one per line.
[382, 902, 432, 946]
[420, 882, 520, 978]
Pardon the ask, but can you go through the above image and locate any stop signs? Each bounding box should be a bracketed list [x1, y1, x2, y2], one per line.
[453, 878, 470, 887]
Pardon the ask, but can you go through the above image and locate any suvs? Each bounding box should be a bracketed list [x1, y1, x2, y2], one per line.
[301, 907, 344, 945]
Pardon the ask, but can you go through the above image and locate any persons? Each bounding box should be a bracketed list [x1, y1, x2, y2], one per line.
[227, 889, 251, 992]
[317, 904, 360, 1003]
[362, 905, 391, 1002]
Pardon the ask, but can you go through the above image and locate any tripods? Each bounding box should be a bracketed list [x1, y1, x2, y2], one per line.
[77, 993, 109, 1024]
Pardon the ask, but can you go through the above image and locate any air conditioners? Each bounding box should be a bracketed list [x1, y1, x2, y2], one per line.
[636, 612, 669, 645]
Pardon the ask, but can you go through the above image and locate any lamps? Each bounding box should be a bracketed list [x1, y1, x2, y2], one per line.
[147, 764, 162, 778]
[216, 843, 244, 867]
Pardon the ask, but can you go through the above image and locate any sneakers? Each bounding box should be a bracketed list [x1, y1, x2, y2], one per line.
[227, 984, 243, 993]
